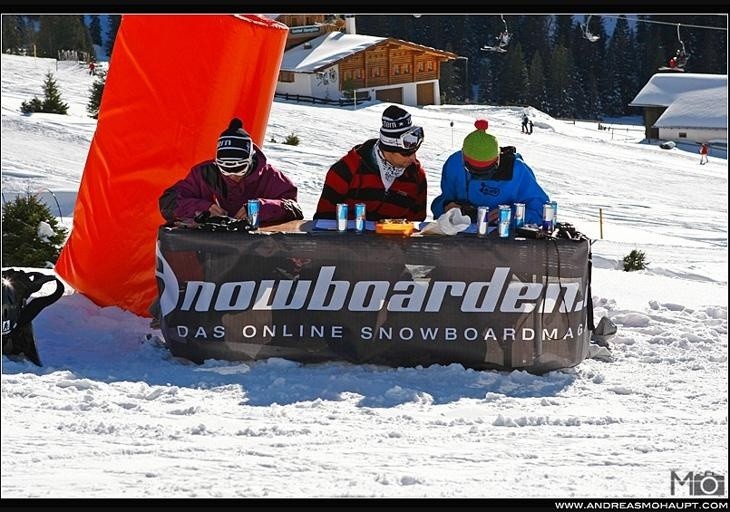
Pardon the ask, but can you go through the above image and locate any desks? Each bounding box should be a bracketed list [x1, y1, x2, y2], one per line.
[154, 218, 596, 378]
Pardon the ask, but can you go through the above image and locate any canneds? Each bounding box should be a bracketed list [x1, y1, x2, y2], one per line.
[477, 206, 490, 240]
[336, 204, 349, 233]
[497, 205, 511, 233]
[354, 203, 367, 235]
[513, 203, 526, 233]
[247, 200, 261, 230]
[499, 209, 512, 238]
[542, 201, 557, 234]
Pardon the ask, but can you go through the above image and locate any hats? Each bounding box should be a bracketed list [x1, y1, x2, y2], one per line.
[461, 120, 499, 169]
[215, 119, 253, 162]
[379, 105, 423, 156]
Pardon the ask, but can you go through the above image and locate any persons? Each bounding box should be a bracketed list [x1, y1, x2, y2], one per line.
[669, 57, 678, 70]
[587, 29, 601, 43]
[430, 120, 550, 227]
[158, 118, 304, 341]
[87, 62, 97, 75]
[520, 114, 529, 134]
[699, 143, 709, 165]
[313, 105, 428, 222]
[528, 121, 534, 133]
[676, 50, 685, 66]
[493, 30, 508, 48]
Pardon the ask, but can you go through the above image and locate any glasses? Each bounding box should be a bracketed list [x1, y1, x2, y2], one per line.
[216, 162, 251, 176]
[399, 126, 425, 152]
